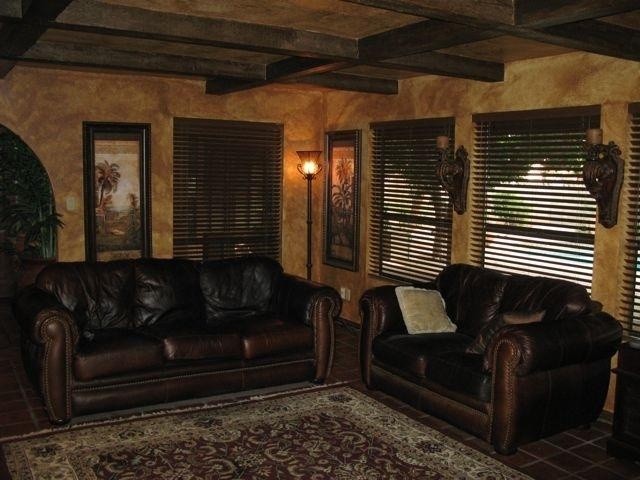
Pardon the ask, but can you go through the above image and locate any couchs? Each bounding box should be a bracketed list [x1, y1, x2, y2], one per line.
[358, 263, 623, 456]
[10, 254, 342, 426]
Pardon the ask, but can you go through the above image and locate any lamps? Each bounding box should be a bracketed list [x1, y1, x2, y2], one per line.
[576, 129, 625, 229]
[295, 150, 323, 281]
[435, 135, 471, 215]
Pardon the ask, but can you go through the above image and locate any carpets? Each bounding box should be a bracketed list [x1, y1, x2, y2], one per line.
[0, 378, 539, 480]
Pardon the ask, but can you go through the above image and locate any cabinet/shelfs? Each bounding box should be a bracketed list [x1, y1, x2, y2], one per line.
[607, 336, 640, 462]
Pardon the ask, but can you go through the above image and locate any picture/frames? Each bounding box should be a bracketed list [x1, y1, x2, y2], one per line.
[81, 119, 152, 263]
[321, 129, 362, 273]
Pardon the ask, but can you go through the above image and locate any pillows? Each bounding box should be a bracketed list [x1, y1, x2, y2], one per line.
[465, 307, 546, 355]
[394, 284, 459, 335]
